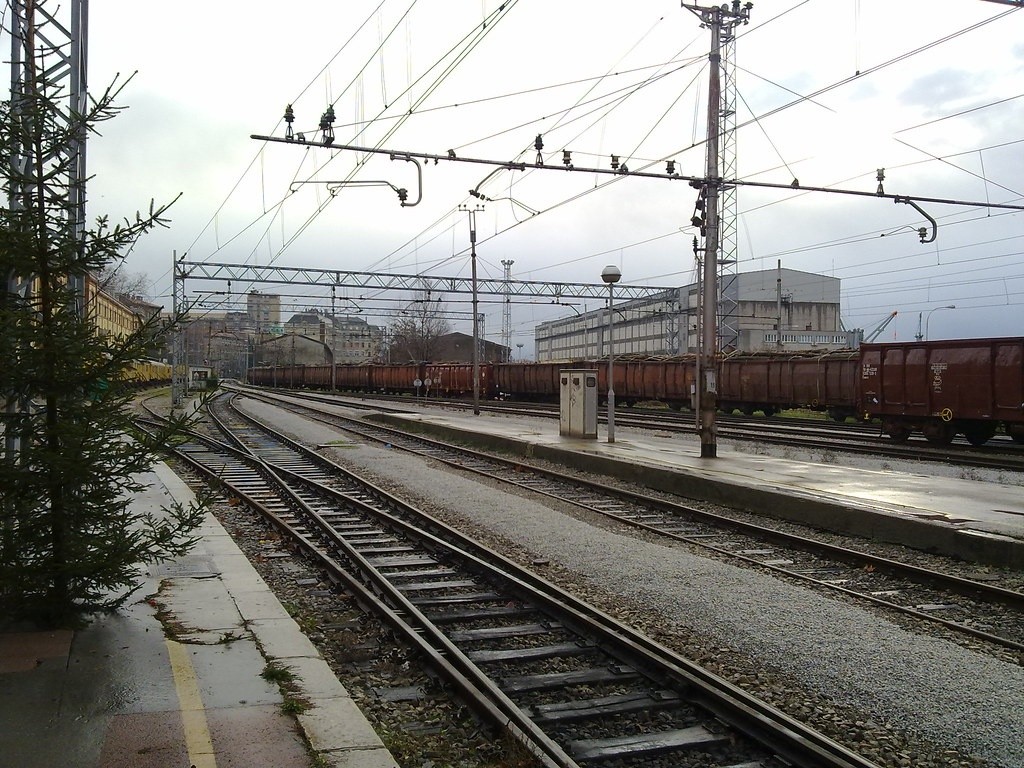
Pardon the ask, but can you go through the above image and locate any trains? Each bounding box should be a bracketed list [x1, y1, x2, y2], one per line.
[246, 335, 1024, 444]
[108, 358, 190, 388]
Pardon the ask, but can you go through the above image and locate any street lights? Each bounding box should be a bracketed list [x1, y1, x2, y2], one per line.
[599, 266, 621, 445]
[501, 260, 515, 363]
[926, 304, 956, 341]
[516, 343, 524, 362]
